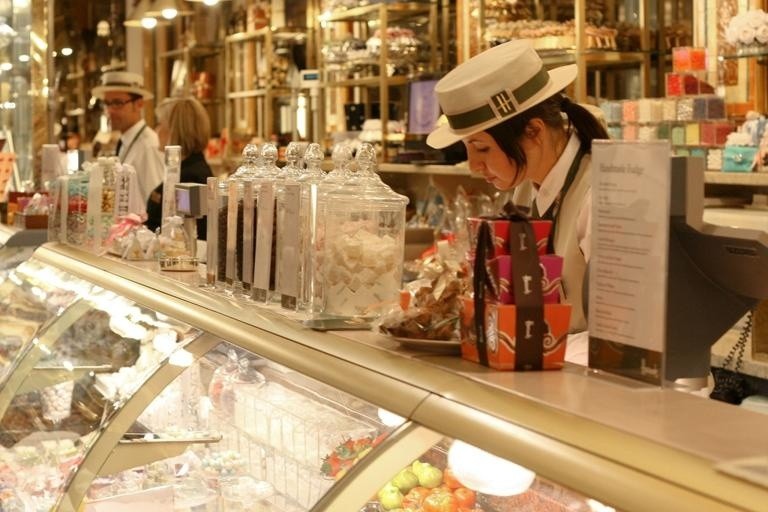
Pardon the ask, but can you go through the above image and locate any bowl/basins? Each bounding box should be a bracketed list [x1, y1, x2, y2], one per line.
[377, 326, 463, 356]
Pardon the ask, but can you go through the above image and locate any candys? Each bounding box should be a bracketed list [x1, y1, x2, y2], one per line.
[65, 169, 405, 321]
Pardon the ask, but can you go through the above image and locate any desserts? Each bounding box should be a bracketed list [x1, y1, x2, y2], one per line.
[0, 299, 373, 512]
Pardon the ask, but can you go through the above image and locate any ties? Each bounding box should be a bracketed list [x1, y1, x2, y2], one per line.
[116, 139, 122, 156]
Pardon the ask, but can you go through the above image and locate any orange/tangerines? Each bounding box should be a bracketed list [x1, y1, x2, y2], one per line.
[402, 468, 486, 512]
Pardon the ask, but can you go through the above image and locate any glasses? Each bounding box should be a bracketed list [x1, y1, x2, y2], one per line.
[101, 98, 131, 108]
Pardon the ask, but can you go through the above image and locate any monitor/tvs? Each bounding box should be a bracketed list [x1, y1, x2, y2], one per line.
[406, 75, 459, 149]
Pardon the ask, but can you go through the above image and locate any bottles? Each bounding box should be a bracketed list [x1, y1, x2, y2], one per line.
[207, 138, 410, 333]
[465, 0, 616, 56]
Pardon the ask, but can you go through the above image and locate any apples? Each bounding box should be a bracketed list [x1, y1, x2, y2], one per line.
[378, 460, 443, 508]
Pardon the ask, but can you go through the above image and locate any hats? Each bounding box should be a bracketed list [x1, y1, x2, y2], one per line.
[91, 72, 155, 101]
[426, 39, 578, 150]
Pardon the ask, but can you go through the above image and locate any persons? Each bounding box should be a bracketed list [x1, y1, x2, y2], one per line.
[133, 95, 215, 242]
[91, 70, 167, 217]
[425, 38, 611, 370]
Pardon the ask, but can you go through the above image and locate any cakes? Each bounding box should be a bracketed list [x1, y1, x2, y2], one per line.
[598, 44, 735, 172]
